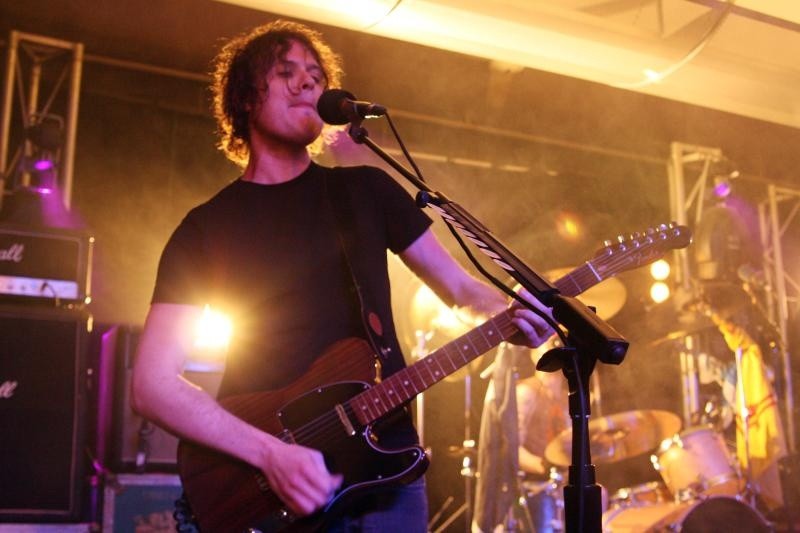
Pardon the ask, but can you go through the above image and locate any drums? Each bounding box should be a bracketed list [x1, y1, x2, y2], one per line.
[610, 480, 670, 506]
[652, 425, 748, 499]
[602, 495, 774, 533]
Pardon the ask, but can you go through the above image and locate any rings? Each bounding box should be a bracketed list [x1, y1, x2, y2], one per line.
[544, 324, 550, 332]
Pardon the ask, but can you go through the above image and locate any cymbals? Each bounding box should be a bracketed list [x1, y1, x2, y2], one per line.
[401, 279, 489, 384]
[631, 283, 752, 354]
[507, 267, 629, 332]
[544, 409, 682, 469]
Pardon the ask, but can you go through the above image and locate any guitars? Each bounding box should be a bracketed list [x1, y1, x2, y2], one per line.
[176, 219, 695, 533]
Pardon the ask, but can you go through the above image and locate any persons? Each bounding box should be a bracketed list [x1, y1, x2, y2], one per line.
[127, 20, 568, 532]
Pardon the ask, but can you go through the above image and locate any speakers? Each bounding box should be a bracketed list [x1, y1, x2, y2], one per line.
[108, 326, 228, 464]
[0, 304, 84, 524]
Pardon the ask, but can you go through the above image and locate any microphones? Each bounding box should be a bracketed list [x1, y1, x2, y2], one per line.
[315, 88, 387, 125]
